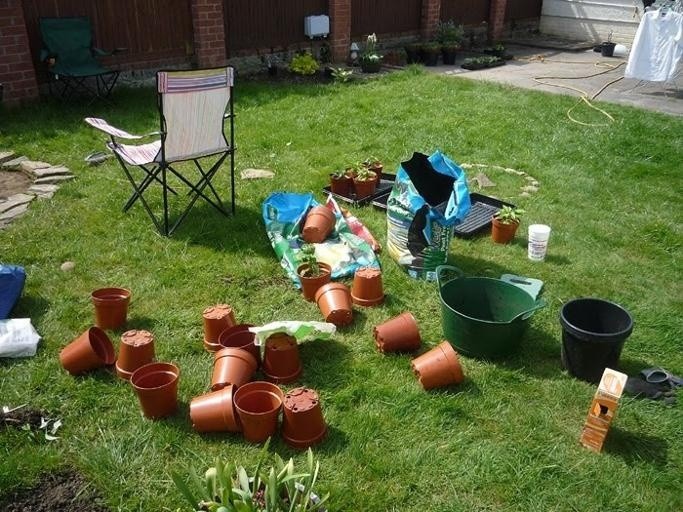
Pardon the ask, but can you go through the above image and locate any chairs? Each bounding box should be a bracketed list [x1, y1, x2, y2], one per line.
[84, 65, 238, 237]
[35, 16, 128, 106]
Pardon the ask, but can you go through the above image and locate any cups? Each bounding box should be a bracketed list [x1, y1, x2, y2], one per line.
[529, 222, 550, 265]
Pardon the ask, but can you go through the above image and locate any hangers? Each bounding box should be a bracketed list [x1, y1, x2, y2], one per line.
[660, 3, 669, 11]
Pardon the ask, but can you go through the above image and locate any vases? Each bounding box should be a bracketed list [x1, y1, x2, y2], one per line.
[55, 267, 463, 446]
[301, 205, 336, 242]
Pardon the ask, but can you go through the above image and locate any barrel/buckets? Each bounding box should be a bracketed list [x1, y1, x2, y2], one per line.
[526, 222, 552, 262]
[559, 297, 635, 384]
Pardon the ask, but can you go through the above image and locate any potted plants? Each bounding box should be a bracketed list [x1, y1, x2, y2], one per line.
[330, 155, 383, 198]
[492, 43, 507, 57]
[295, 242, 333, 302]
[491, 203, 525, 244]
[421, 20, 459, 66]
[360, 30, 384, 73]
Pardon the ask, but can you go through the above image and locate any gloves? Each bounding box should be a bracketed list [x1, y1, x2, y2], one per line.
[623, 365, 682, 405]
